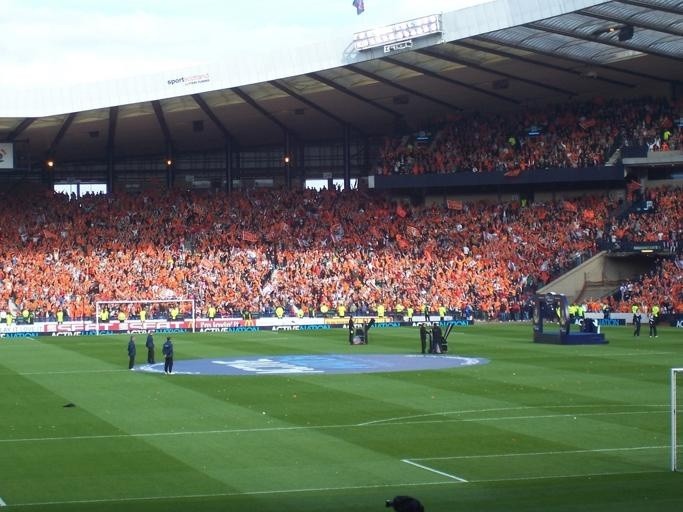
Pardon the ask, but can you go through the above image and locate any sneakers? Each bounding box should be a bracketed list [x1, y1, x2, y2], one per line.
[164, 371, 176, 375]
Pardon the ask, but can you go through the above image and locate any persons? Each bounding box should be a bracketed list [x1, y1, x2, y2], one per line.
[1, 89, 682, 327]
[431, 323, 443, 353]
[631, 305, 641, 336]
[161, 335, 174, 375]
[144, 331, 155, 364]
[348, 324, 368, 345]
[420, 323, 429, 354]
[127, 334, 136, 370]
[646, 311, 658, 339]
